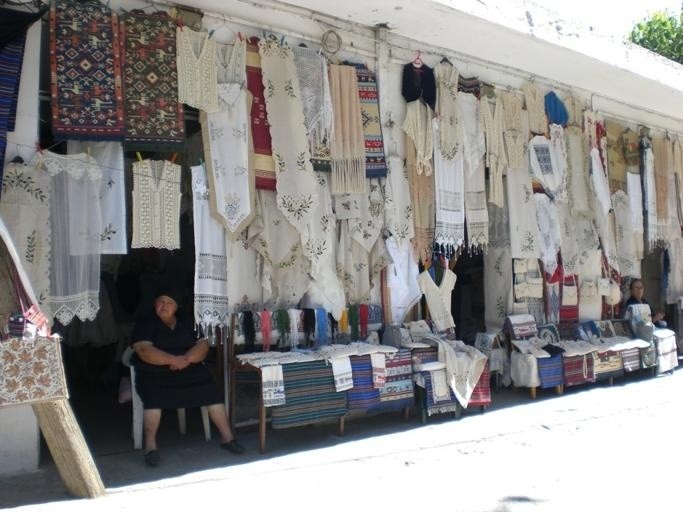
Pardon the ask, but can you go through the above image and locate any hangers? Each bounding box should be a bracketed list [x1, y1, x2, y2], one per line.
[427, 252, 445, 271]
[0, 1, 671, 167]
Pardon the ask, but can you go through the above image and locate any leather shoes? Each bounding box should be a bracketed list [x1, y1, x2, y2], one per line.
[143, 447, 161, 468]
[219, 438, 245, 455]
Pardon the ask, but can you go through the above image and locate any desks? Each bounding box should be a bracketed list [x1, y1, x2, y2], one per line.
[411, 347, 491, 425]
[512, 339, 655, 400]
[229, 341, 414, 454]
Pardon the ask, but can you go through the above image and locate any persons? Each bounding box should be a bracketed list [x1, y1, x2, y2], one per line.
[626, 278, 668, 327]
[126, 288, 248, 467]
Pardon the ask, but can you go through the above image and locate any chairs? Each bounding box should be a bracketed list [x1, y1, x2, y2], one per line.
[121, 346, 211, 450]
